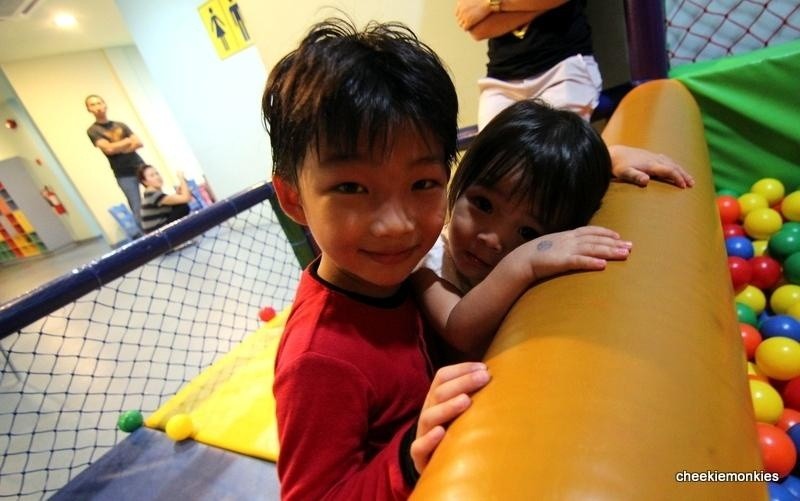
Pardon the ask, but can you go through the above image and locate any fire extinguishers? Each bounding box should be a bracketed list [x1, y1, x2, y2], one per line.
[40, 185, 65, 215]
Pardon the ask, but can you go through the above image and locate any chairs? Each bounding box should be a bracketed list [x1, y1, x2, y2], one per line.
[108, 203, 143, 242]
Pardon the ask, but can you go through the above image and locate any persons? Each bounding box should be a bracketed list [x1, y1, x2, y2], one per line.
[415, 100, 694, 357]
[85, 94, 144, 233]
[137, 165, 190, 236]
[454, 0, 602, 130]
[717, 178, 800, 500]
[261, 18, 492, 501]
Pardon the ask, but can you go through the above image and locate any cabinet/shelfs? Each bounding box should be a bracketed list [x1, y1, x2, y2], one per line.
[0, 156, 77, 268]
